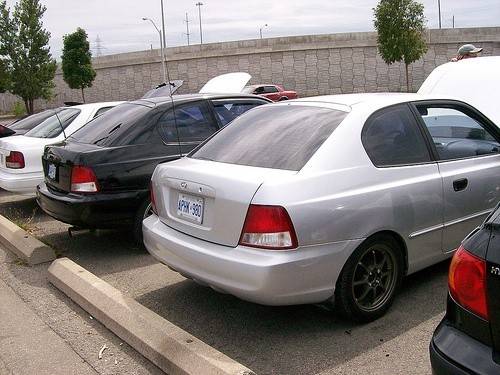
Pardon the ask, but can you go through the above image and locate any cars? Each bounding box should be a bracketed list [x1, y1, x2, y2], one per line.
[0, 56, 500, 375]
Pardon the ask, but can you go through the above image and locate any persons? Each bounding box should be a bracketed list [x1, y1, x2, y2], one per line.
[451, 42, 491, 139]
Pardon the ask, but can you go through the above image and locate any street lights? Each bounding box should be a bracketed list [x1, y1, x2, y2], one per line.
[142, 17, 167, 83]
[195, 1, 204, 44]
[260, 23, 268, 38]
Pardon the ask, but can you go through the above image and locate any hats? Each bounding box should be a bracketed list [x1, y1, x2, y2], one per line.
[457, 44, 483, 57]
